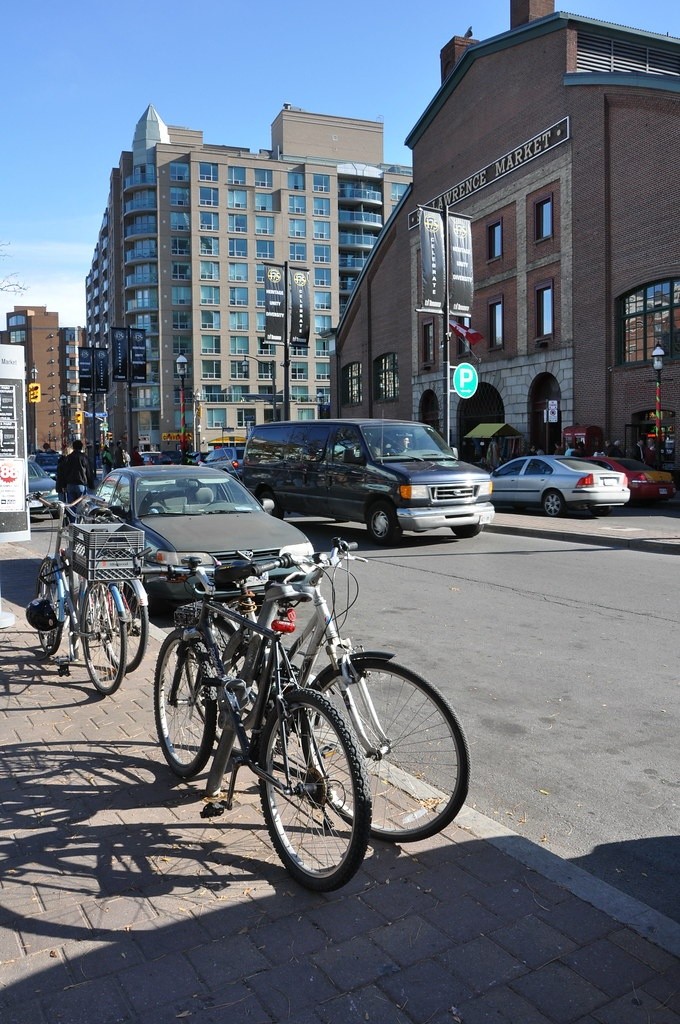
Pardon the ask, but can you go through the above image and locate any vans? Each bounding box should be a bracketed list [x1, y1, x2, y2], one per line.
[241, 419, 496, 544]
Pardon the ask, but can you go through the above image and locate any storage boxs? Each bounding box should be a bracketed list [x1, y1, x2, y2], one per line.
[70, 523, 144, 581]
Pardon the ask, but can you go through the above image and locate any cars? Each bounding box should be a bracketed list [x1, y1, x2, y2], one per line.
[90, 463, 319, 617]
[26, 460, 65, 520]
[578, 455, 677, 505]
[136, 445, 247, 478]
[26, 452, 65, 480]
[486, 453, 630, 517]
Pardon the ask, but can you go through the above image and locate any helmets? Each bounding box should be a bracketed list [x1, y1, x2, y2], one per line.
[25, 598, 63, 631]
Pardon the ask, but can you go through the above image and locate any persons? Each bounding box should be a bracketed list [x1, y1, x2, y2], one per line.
[43, 443, 55, 453]
[131, 446, 145, 466]
[389, 436, 412, 455]
[55, 440, 95, 526]
[100, 444, 113, 481]
[553, 439, 624, 457]
[645, 437, 658, 471]
[122, 450, 131, 467]
[114, 440, 125, 470]
[633, 438, 646, 464]
[526, 444, 544, 456]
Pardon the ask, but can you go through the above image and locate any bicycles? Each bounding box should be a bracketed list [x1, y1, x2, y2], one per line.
[151, 538, 472, 895]
[21, 489, 150, 696]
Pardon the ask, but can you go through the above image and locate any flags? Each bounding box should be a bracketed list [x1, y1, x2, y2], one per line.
[449, 319, 485, 346]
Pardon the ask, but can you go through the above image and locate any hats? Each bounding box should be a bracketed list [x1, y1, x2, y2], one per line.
[614, 441, 621, 447]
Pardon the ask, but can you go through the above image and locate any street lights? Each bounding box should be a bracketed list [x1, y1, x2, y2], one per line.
[241, 355, 277, 421]
[653, 341, 665, 471]
[59, 392, 69, 451]
[175, 354, 190, 466]
[30, 363, 37, 449]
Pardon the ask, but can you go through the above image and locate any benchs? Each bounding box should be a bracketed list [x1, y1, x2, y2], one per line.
[152, 490, 193, 506]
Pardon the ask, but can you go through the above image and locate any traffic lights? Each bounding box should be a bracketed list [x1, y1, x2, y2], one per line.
[29, 383, 41, 403]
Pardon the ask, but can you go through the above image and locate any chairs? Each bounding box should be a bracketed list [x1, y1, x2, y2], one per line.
[191, 488, 214, 503]
